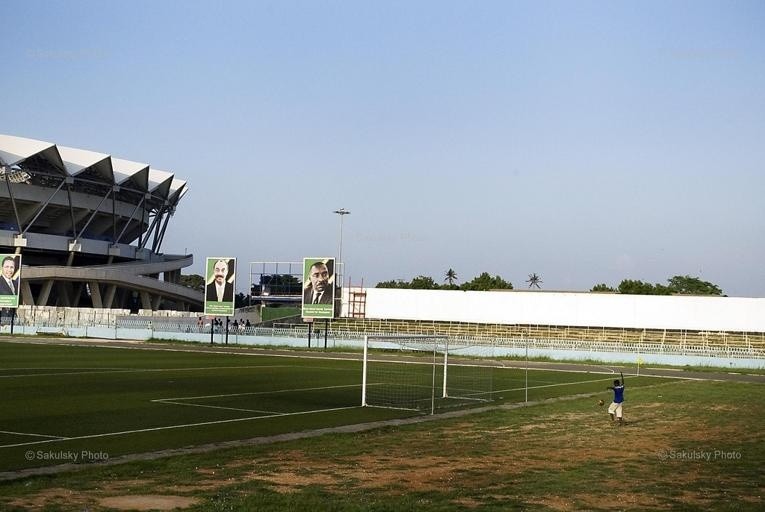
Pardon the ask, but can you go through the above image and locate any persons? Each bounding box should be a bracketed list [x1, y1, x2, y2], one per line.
[0, 256, 18, 294]
[304, 262, 333, 305]
[204, 317, 251, 335]
[197, 317, 203, 333]
[207, 260, 233, 301]
[606, 370, 625, 424]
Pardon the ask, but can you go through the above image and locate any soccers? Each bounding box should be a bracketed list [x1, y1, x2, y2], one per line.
[598, 400, 604, 406]
